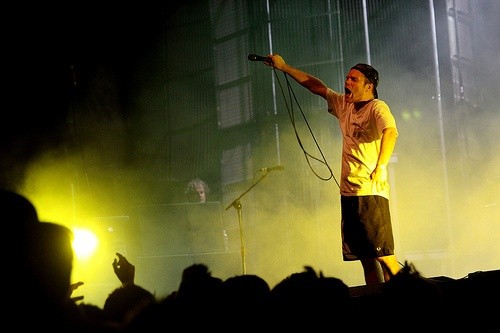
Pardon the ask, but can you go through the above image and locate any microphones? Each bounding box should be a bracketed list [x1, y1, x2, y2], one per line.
[248, 54, 272, 63]
[259, 165, 284, 171]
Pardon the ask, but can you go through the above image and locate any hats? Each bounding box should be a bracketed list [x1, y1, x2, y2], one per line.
[351, 63, 379, 99]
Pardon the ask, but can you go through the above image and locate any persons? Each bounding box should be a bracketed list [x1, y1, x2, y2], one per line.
[266, 54, 400, 285]
[0, 191, 500, 333]
